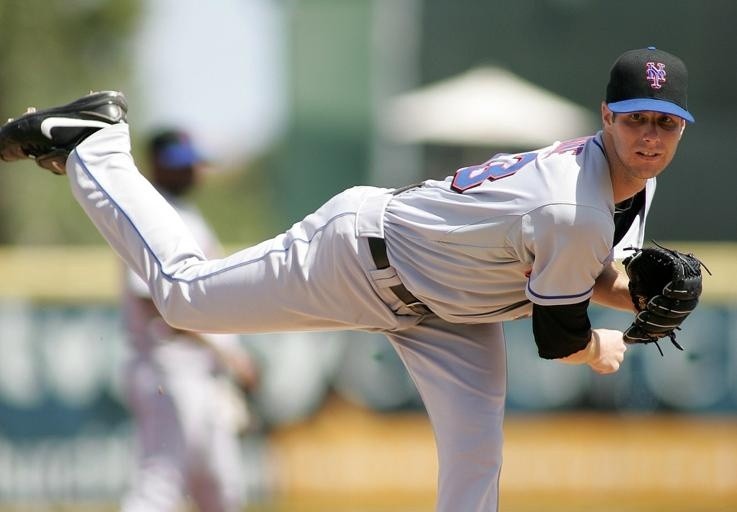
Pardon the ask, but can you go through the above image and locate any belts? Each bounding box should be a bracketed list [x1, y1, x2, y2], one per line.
[367, 236, 434, 315]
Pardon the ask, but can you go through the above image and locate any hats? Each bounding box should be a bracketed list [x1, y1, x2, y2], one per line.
[606, 47, 697, 124]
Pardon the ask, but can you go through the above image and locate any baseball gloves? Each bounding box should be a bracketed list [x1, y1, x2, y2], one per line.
[624, 237, 710, 356]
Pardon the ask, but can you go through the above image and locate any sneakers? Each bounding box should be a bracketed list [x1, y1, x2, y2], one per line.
[0, 89, 128, 175]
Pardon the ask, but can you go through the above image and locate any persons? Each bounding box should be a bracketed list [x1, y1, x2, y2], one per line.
[1, 34, 702, 512]
[102, 116, 262, 512]
